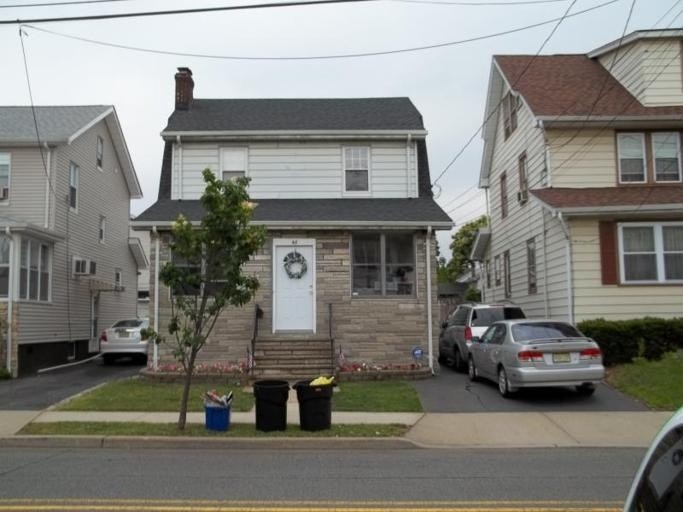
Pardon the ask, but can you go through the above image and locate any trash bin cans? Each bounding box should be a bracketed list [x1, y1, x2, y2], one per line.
[203, 403, 230, 431]
[253, 379, 337, 432]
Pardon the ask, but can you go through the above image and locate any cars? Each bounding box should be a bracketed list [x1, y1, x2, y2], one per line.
[98, 317, 150, 364]
[439, 298, 526, 373]
[467, 317, 605, 399]
[624, 404, 683, 512]
